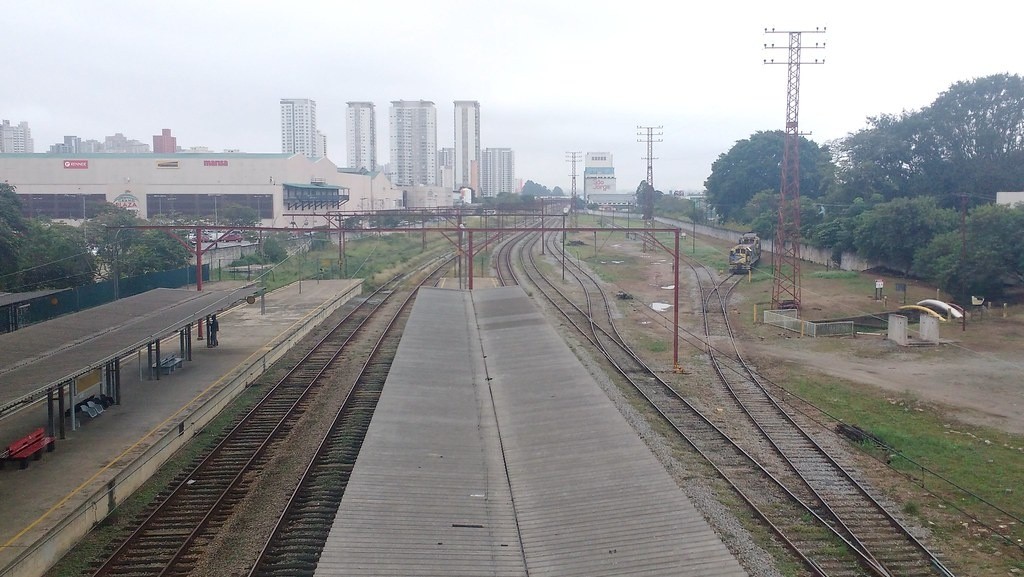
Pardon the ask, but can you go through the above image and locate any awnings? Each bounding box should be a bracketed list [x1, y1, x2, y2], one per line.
[284, 182, 349, 189]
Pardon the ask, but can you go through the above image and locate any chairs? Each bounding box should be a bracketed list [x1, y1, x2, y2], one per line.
[100, 394, 115, 407]
[87, 401, 104, 415]
[94, 398, 110, 410]
[80, 405, 97, 421]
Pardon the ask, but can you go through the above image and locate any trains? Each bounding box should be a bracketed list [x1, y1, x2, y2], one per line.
[729, 232, 761, 275]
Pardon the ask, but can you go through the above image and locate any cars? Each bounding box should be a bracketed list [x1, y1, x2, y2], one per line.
[173, 219, 243, 245]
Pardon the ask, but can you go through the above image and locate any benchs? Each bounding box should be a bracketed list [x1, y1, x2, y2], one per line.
[65, 395, 95, 418]
[152, 352, 184, 376]
[0, 427, 57, 470]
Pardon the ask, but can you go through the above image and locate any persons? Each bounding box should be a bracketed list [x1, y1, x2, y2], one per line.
[209, 314, 219, 347]
